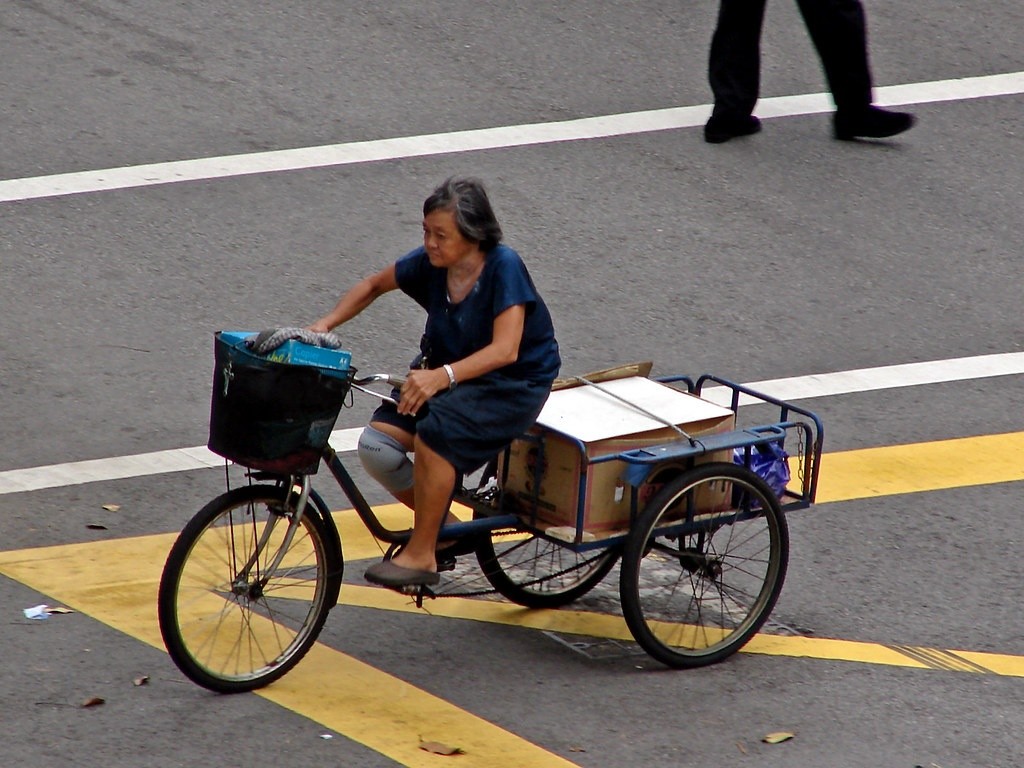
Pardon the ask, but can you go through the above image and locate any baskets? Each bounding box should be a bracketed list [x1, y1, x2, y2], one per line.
[208, 330, 358, 475]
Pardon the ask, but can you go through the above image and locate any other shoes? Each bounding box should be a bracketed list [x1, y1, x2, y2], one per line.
[833, 105, 912, 140]
[705, 116, 760, 143]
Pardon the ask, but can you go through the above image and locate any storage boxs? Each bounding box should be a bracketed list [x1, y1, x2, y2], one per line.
[496, 360, 736, 536]
[218, 329, 350, 462]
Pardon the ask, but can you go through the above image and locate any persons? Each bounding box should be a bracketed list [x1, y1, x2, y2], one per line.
[701, 0, 916, 145]
[304, 173, 561, 589]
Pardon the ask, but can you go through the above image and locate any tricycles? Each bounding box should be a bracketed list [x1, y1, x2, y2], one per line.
[155, 328, 825, 695]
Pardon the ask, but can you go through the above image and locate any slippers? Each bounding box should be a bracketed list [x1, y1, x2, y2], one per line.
[364, 562, 440, 586]
[435, 540, 475, 559]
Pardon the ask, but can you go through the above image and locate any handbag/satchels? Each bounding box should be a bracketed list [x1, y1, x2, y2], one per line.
[732, 441, 791, 510]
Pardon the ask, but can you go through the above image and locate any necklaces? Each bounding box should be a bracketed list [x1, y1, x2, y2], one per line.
[451, 257, 484, 287]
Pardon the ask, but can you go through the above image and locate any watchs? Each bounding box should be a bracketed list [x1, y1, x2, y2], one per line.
[443, 363, 457, 390]
